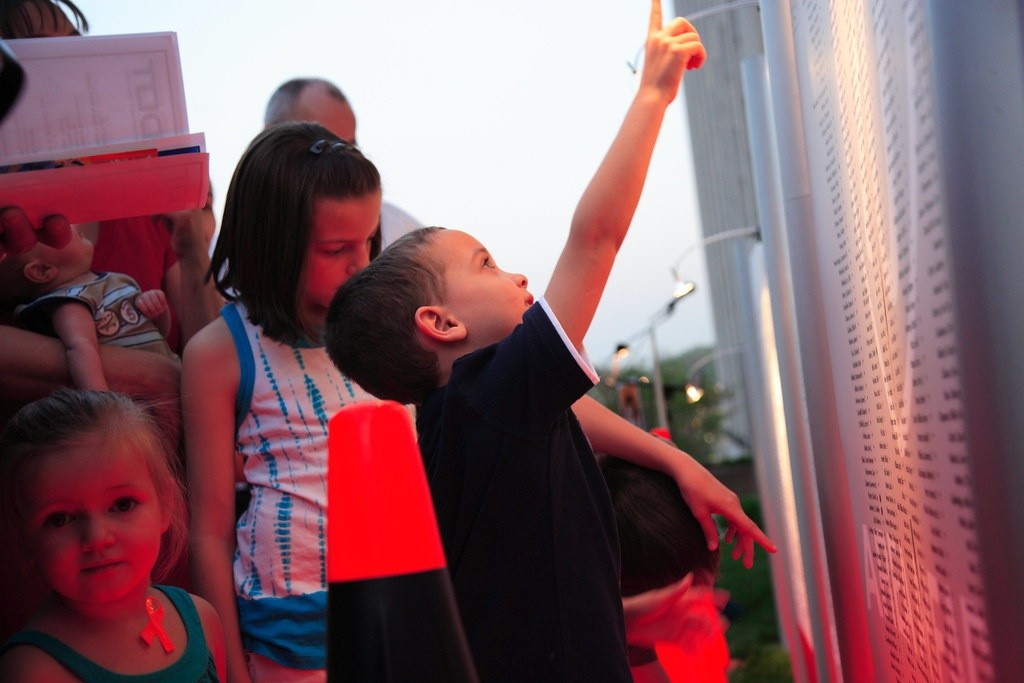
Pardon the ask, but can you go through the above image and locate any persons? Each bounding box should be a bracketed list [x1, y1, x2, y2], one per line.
[597, 455, 731, 682]
[0, 0, 425, 682]
[324, 0, 778, 683]
